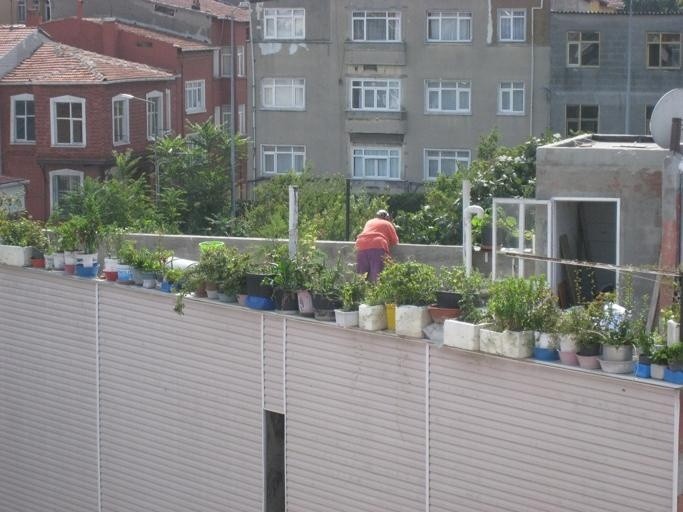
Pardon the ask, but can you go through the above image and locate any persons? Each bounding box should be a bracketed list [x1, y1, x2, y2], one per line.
[353, 209, 400, 287]
